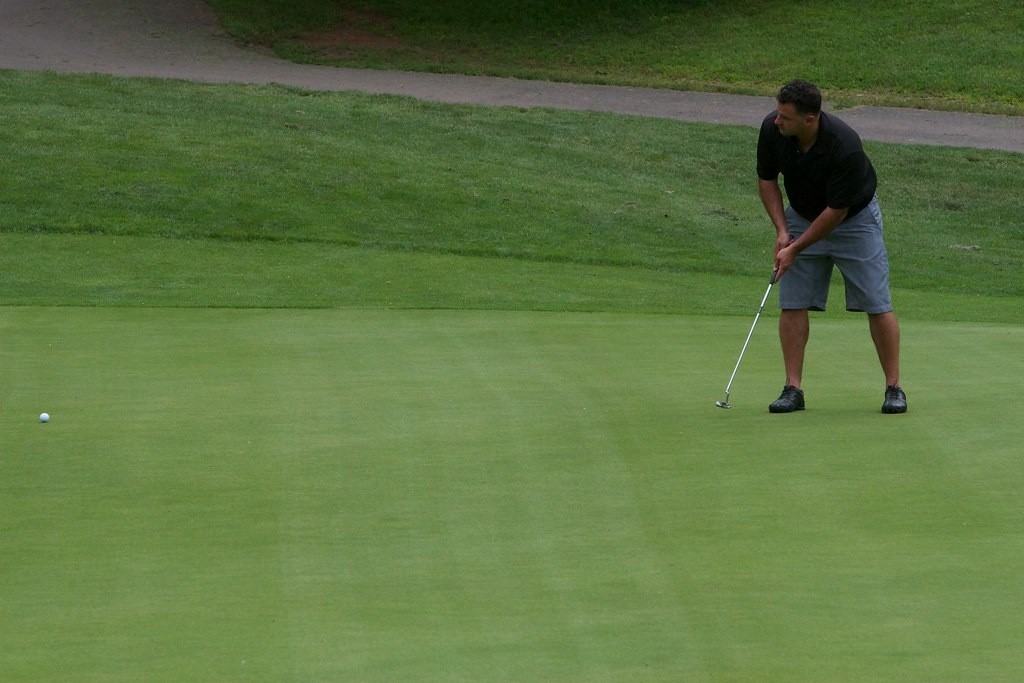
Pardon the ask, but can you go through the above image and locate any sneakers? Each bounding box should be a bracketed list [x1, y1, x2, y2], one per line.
[882, 378, 907, 413]
[769, 377, 805, 412]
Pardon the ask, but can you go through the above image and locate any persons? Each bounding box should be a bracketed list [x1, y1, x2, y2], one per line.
[756, 79, 908, 414]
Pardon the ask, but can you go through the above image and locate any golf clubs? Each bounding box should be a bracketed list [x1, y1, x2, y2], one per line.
[715, 233, 795, 409]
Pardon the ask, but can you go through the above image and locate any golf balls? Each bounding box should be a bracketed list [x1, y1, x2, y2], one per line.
[40, 412, 50, 422]
